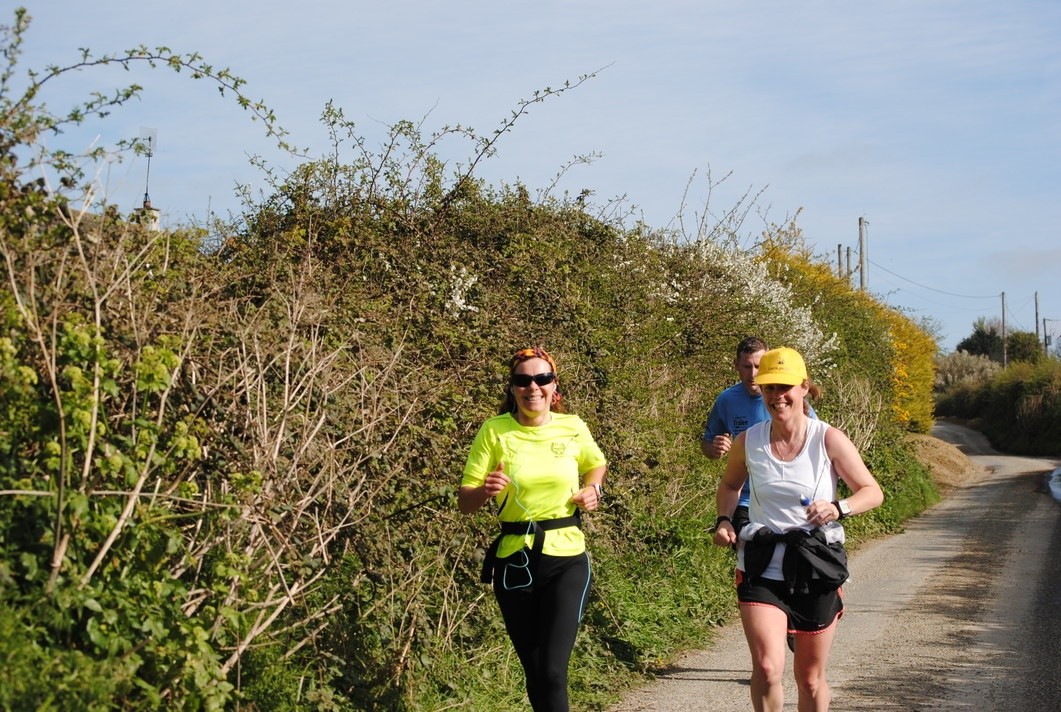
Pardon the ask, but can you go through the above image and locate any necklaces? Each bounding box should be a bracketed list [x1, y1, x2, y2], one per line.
[770, 421, 804, 463]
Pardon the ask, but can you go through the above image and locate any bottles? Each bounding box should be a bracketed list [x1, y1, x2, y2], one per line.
[798, 495, 834, 532]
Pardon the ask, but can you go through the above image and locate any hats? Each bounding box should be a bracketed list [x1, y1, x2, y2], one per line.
[754, 347, 808, 386]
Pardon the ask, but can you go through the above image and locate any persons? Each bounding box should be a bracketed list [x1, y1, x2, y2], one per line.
[459, 345, 609, 712]
[711, 344, 888, 712]
[700, 337, 819, 656]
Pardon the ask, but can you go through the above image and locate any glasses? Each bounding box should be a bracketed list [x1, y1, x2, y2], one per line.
[512, 372, 556, 387]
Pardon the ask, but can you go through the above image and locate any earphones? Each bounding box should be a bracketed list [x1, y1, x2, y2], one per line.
[509, 385, 514, 395]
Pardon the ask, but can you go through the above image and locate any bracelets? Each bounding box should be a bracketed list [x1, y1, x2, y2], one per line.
[714, 517, 729, 527]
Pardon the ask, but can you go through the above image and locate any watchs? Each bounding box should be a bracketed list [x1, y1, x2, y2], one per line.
[828, 499, 850, 522]
[588, 481, 602, 505]
